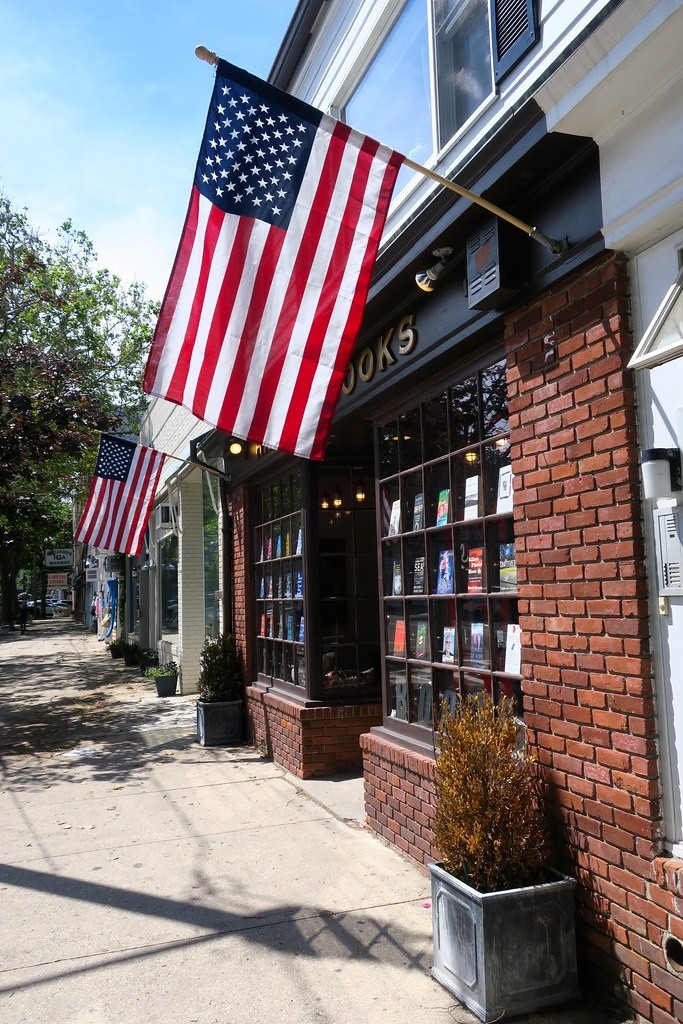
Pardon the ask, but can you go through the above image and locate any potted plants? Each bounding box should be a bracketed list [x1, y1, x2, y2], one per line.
[194, 633, 242, 747]
[105, 637, 127, 659]
[123, 642, 143, 665]
[426, 689, 578, 1022]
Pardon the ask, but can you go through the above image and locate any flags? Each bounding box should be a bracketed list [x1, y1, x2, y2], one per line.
[144, 62, 407, 458]
[73, 433, 166, 555]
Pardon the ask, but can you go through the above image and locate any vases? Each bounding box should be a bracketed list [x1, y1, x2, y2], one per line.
[154, 675, 178, 697]
[139, 658, 158, 675]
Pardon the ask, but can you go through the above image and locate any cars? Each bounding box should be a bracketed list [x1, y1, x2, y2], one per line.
[27, 600, 53, 617]
[57, 600, 72, 604]
[53, 602, 72, 609]
[45, 599, 59, 606]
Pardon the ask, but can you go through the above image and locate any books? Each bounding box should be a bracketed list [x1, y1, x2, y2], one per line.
[393, 620, 405, 657]
[499, 543, 519, 593]
[417, 622, 427, 660]
[467, 547, 486, 594]
[412, 493, 424, 531]
[392, 560, 403, 596]
[442, 627, 457, 663]
[413, 557, 426, 595]
[258, 529, 305, 643]
[437, 550, 454, 594]
[436, 489, 451, 526]
[464, 475, 483, 520]
[497, 465, 515, 515]
[470, 623, 490, 668]
[388, 500, 401, 535]
[504, 625, 522, 674]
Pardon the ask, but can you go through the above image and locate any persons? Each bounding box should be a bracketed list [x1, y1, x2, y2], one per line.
[20, 601, 28, 635]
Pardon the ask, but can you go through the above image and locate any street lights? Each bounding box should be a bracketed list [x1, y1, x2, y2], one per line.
[23, 573, 27, 591]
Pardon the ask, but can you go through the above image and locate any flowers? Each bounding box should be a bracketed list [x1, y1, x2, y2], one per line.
[144, 661, 181, 680]
[138, 648, 159, 660]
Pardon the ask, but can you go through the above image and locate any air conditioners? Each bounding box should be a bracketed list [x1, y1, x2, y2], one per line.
[155, 502, 178, 530]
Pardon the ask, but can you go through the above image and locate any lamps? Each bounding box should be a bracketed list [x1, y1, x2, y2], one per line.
[637, 448, 682, 501]
[132, 564, 140, 577]
[229, 438, 242, 454]
[416, 247, 452, 293]
[105, 555, 119, 572]
